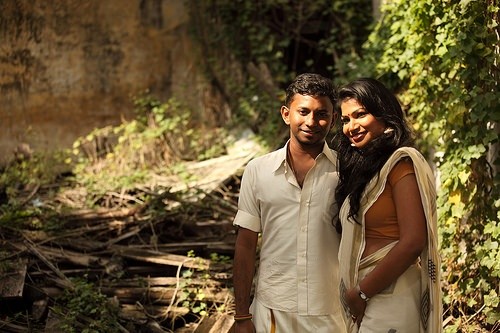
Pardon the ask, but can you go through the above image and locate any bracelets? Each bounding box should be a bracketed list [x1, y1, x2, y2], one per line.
[234, 313, 253, 321]
[356, 284, 370, 301]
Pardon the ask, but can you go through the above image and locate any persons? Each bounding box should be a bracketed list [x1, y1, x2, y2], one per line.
[330, 78, 442, 333]
[228, 73, 347, 333]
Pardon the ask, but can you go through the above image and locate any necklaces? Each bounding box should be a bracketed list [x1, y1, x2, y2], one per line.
[288, 145, 306, 186]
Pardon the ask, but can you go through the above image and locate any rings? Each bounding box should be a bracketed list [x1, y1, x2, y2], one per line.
[352, 315, 356, 319]
